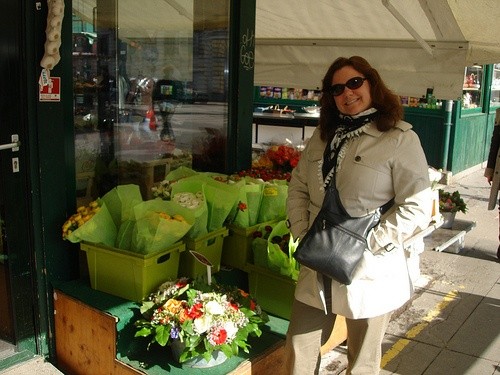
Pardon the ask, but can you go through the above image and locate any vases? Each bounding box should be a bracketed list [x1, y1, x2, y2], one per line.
[441, 211, 456, 229]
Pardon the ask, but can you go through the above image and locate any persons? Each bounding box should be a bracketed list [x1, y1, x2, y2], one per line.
[484, 108, 500, 260]
[284, 56, 433, 375]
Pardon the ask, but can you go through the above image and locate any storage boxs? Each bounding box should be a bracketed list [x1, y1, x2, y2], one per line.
[221, 219, 278, 270]
[172, 339, 227, 368]
[80, 241, 186, 305]
[179, 226, 230, 280]
[243, 263, 299, 321]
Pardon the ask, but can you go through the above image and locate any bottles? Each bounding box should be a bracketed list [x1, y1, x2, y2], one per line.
[427, 94, 442, 109]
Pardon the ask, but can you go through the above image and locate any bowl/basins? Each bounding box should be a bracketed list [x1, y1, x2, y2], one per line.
[305, 107, 320, 113]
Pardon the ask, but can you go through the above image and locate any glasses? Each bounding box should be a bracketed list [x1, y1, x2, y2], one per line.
[328, 77, 368, 96]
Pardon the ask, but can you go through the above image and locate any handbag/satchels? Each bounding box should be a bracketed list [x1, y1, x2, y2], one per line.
[292, 181, 383, 285]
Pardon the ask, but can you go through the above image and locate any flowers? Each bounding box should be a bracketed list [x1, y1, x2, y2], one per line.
[439, 189, 469, 214]
[62, 143, 303, 363]
